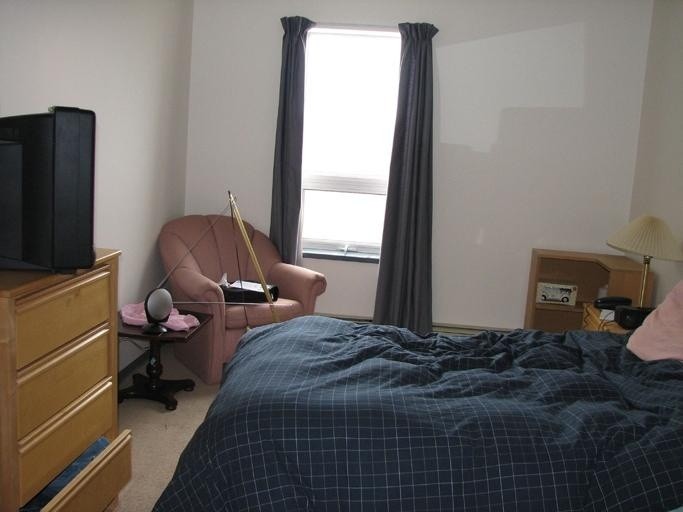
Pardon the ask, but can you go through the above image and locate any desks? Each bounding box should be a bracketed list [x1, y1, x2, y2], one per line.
[117, 304, 214, 410]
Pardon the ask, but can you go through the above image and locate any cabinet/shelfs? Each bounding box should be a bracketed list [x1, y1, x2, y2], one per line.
[0, 247, 134, 512]
[523, 248, 655, 330]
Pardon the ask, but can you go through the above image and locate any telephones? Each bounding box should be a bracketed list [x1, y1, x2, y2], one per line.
[593, 296, 633, 309]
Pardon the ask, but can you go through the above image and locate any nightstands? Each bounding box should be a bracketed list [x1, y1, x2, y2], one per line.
[580, 302, 649, 334]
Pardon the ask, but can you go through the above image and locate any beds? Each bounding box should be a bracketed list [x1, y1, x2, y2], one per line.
[147, 272, 683, 512]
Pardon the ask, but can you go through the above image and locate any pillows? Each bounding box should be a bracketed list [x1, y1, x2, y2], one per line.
[626, 278, 683, 361]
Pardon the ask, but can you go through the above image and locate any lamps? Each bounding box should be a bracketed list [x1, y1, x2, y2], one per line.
[605, 216, 683, 307]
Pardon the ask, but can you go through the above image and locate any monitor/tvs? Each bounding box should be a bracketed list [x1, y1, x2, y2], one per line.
[0, 106, 96, 275]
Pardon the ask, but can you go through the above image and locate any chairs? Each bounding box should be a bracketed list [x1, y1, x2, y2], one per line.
[158, 214, 327, 386]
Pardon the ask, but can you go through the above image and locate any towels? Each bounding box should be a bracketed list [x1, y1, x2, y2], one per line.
[122, 300, 199, 331]
